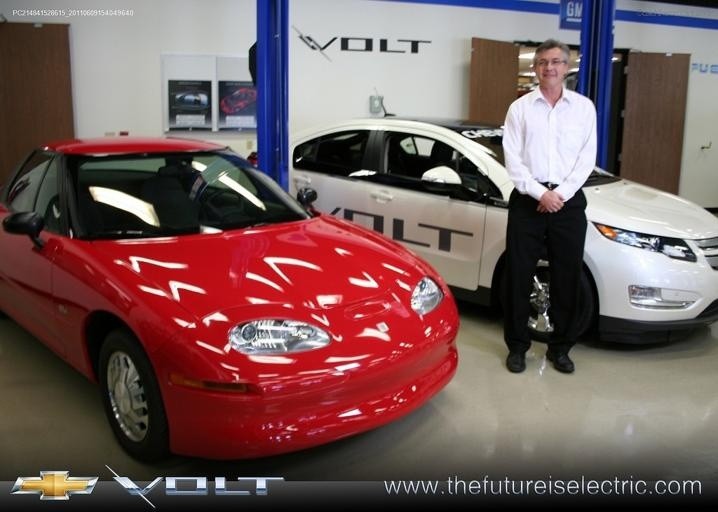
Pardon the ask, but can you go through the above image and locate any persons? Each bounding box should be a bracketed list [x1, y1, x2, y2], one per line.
[502, 39, 597, 373]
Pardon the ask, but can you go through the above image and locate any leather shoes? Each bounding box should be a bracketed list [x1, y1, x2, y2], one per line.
[546, 350, 575, 373]
[506, 352, 526, 372]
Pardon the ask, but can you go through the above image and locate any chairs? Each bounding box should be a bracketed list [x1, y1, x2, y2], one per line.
[76, 174, 195, 231]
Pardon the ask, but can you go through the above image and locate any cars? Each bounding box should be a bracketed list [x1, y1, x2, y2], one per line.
[0, 136, 461, 465]
[243, 115, 718, 343]
[220, 88, 257, 114]
[173, 92, 209, 112]
